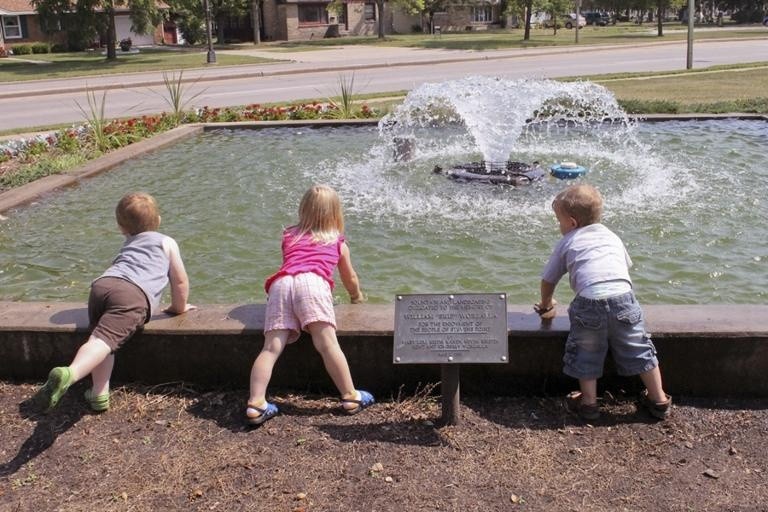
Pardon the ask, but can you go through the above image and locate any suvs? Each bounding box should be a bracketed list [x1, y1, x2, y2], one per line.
[580, 11, 609, 26]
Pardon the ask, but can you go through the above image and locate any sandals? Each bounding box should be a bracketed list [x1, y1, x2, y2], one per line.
[247, 400, 279, 425]
[35, 366, 71, 414]
[83, 387, 110, 411]
[341, 390, 374, 415]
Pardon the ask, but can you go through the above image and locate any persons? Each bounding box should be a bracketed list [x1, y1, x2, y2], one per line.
[244, 184, 375, 427]
[30, 192, 197, 414]
[535, 184, 673, 420]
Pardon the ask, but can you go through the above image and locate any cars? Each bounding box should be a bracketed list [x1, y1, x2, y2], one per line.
[542, 14, 587, 30]
[761, 16, 768, 26]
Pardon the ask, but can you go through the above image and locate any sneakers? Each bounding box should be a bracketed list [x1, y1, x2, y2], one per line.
[565, 391, 601, 420]
[640, 388, 672, 418]
